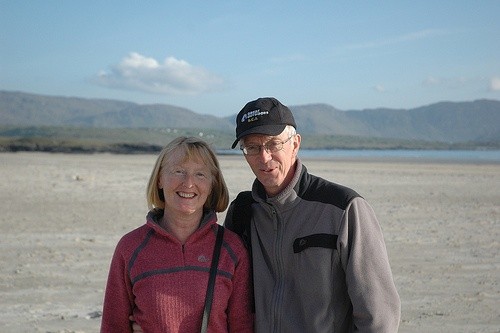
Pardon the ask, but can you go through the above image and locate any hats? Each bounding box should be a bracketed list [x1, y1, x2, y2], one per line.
[231, 97, 297, 149]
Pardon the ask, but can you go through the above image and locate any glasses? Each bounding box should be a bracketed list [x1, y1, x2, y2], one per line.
[239, 134, 295, 155]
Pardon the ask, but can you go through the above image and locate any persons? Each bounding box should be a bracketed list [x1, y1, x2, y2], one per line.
[100, 135, 250, 333]
[223, 97, 402, 333]
